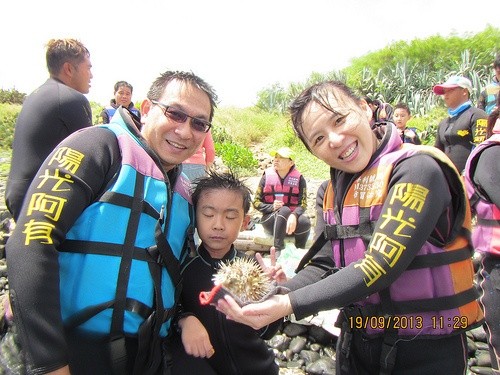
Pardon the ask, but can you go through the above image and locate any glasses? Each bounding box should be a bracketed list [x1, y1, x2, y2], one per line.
[151, 100, 211, 133]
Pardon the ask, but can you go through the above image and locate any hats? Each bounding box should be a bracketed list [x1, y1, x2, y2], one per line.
[433, 75, 472, 98]
[269, 147, 296, 163]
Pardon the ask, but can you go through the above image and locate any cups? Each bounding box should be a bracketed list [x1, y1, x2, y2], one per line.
[275, 194, 284, 201]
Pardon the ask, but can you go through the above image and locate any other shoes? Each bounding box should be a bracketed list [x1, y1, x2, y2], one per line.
[273, 249, 281, 262]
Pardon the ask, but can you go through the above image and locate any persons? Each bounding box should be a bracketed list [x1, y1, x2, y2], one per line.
[358, 54, 500, 375]
[250, 145, 311, 263]
[214, 79, 484, 375]
[5, 37, 94, 226]
[96, 78, 216, 252]
[5, 69, 219, 375]
[172, 169, 288, 375]
